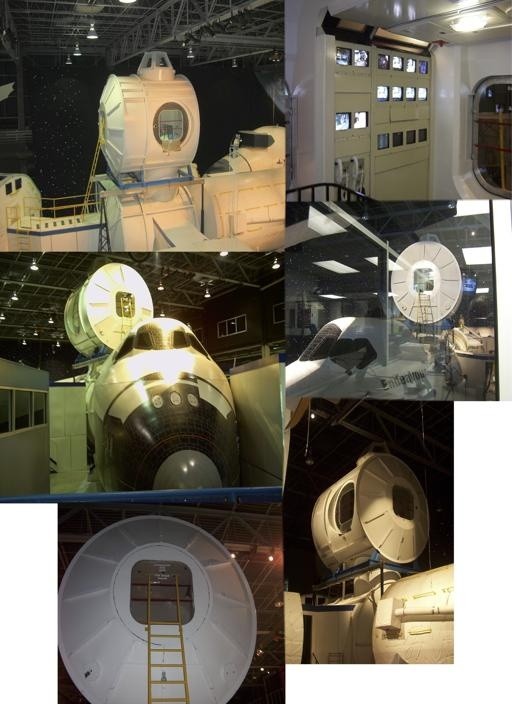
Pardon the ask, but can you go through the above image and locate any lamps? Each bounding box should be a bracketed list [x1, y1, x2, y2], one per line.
[271, 257, 280, 270]
[30, 258, 39, 270]
[33, 330, 38, 336]
[0, 313, 5, 320]
[158, 282, 164, 291]
[232, 58, 238, 68]
[73, 43, 82, 57]
[65, 56, 73, 65]
[204, 289, 211, 298]
[187, 47, 194, 59]
[21, 340, 27, 345]
[56, 341, 60, 347]
[219, 251, 228, 256]
[48, 317, 54, 324]
[87, 23, 99, 39]
[160, 310, 166, 317]
[11, 291, 18, 301]
